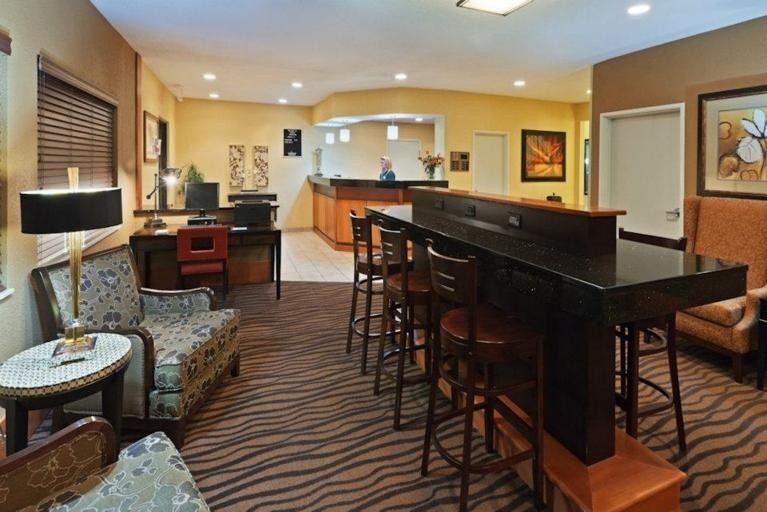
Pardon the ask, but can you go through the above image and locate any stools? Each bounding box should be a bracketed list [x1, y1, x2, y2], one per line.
[270, 205, 283, 221]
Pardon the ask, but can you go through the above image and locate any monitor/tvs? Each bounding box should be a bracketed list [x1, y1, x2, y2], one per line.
[183, 181, 221, 218]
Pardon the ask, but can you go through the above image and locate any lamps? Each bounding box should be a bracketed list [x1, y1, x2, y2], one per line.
[18, 166, 124, 357]
[325, 124, 334, 145]
[454, 0, 536, 17]
[339, 121, 350, 143]
[386, 118, 399, 141]
[144, 167, 183, 228]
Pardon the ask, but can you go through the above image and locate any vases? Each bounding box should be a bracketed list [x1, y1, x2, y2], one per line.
[426, 171, 435, 181]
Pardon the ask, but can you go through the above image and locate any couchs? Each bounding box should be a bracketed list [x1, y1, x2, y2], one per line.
[677, 193, 767, 384]
[1, 416, 211, 512]
[28, 243, 242, 448]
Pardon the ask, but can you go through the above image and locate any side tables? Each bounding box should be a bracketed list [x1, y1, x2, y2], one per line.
[0, 331, 132, 463]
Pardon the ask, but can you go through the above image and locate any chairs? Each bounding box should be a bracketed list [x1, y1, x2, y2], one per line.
[346, 208, 415, 377]
[372, 216, 449, 430]
[240, 190, 260, 202]
[419, 235, 549, 511]
[176, 225, 229, 300]
[613, 228, 691, 452]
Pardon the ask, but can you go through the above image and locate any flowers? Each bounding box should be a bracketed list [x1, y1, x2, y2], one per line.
[416, 150, 443, 170]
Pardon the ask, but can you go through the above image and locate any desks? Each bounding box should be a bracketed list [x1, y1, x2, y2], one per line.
[306, 175, 447, 252]
[128, 220, 282, 300]
[361, 186, 747, 512]
[226, 192, 279, 202]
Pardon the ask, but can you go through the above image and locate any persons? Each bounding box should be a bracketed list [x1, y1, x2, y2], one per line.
[379, 155, 395, 181]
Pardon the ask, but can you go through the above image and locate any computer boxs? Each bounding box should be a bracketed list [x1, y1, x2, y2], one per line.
[186, 218, 218, 226]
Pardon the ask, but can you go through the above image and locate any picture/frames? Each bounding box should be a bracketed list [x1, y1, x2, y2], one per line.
[143, 110, 159, 163]
[520, 128, 566, 183]
[695, 85, 767, 201]
